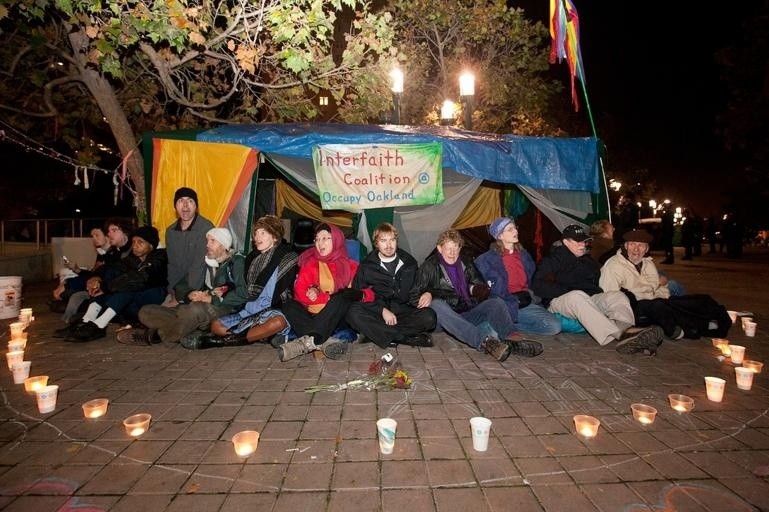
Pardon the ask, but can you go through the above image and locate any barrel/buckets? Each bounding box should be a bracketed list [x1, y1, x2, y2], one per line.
[0, 276, 23, 319]
[60, 267, 88, 285]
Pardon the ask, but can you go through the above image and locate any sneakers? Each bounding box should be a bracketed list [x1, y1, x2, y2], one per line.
[615, 325, 665, 354]
[322, 336, 350, 360]
[481, 335, 511, 362]
[503, 333, 545, 358]
[276, 335, 317, 361]
[356, 331, 372, 345]
[406, 331, 434, 347]
[115, 328, 154, 346]
[271, 334, 288, 348]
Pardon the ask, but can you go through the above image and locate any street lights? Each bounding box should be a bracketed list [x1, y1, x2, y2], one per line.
[439, 99, 456, 130]
[458, 72, 478, 130]
[387, 66, 403, 125]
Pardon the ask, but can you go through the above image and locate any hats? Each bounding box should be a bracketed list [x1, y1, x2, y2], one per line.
[206, 227, 232, 251]
[622, 229, 654, 243]
[133, 226, 161, 248]
[488, 216, 512, 239]
[173, 186, 199, 209]
[561, 224, 594, 242]
[253, 214, 285, 243]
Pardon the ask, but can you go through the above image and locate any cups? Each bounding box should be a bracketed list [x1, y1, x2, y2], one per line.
[19, 315, 27, 329]
[35, 385, 58, 414]
[21, 308, 32, 322]
[735, 367, 754, 390]
[729, 345, 745, 363]
[9, 339, 28, 348]
[11, 323, 22, 335]
[727, 310, 737, 326]
[13, 361, 31, 385]
[470, 416, 492, 451]
[741, 317, 752, 328]
[9, 343, 24, 353]
[747, 323, 757, 335]
[705, 376, 725, 403]
[6, 352, 24, 370]
[375, 418, 397, 453]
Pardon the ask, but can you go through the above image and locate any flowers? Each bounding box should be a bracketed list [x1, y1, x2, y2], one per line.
[304, 358, 413, 395]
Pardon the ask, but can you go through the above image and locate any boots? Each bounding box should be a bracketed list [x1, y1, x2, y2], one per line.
[198, 331, 250, 350]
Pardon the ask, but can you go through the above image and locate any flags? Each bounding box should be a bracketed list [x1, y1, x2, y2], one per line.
[550, 0, 587, 113]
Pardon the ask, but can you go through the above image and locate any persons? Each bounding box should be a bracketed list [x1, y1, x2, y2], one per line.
[530, 225, 664, 356]
[695, 215, 743, 257]
[197, 215, 299, 348]
[680, 211, 693, 260]
[409, 230, 544, 362]
[469, 217, 562, 337]
[656, 215, 675, 265]
[589, 219, 616, 268]
[599, 229, 667, 286]
[278, 223, 375, 362]
[52, 226, 167, 344]
[344, 222, 438, 349]
[621, 203, 639, 235]
[598, 228, 703, 341]
[117, 226, 247, 346]
[45, 225, 110, 304]
[52, 220, 133, 313]
[168, 187, 215, 291]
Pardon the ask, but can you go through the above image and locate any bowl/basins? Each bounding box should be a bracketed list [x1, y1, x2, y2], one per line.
[574, 415, 600, 437]
[123, 413, 151, 437]
[83, 398, 109, 419]
[712, 337, 728, 350]
[232, 430, 260, 457]
[24, 375, 49, 396]
[744, 360, 764, 373]
[630, 404, 658, 423]
[668, 393, 694, 412]
[11, 332, 27, 339]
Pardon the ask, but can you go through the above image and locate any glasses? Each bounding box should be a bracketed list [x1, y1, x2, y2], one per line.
[253, 230, 269, 237]
[313, 236, 333, 243]
[502, 224, 519, 233]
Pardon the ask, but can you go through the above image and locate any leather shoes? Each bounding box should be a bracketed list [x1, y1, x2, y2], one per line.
[52, 319, 86, 338]
[65, 321, 109, 343]
